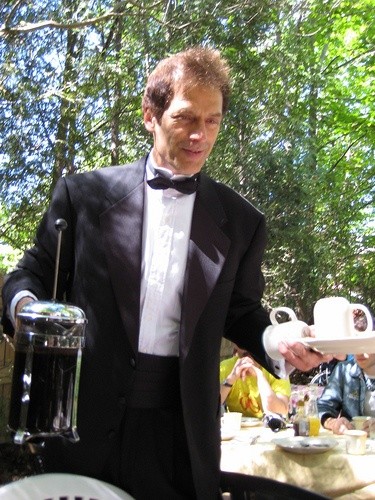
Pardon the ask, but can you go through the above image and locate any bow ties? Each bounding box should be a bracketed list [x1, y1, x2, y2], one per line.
[146, 176, 200, 195]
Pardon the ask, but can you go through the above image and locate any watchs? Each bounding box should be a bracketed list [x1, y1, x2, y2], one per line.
[222, 381, 234, 391]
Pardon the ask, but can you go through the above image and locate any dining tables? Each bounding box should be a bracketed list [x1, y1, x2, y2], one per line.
[218, 424, 375, 500]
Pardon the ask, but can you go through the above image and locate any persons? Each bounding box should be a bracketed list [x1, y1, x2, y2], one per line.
[222, 303, 375, 434]
[0, 47, 347, 497]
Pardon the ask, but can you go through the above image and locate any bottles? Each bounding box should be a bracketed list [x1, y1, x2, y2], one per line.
[293, 391, 310, 436]
[308, 384, 320, 436]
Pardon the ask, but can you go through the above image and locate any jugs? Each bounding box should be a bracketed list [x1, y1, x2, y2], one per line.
[5, 219, 89, 445]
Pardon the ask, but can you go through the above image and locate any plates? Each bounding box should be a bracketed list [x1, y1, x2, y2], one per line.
[303, 331, 375, 353]
[272, 436, 338, 454]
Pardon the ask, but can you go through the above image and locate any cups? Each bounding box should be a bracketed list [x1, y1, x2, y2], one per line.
[351, 416, 371, 430]
[344, 430, 367, 455]
[220, 413, 242, 440]
[314, 297, 374, 339]
[263, 308, 311, 361]
